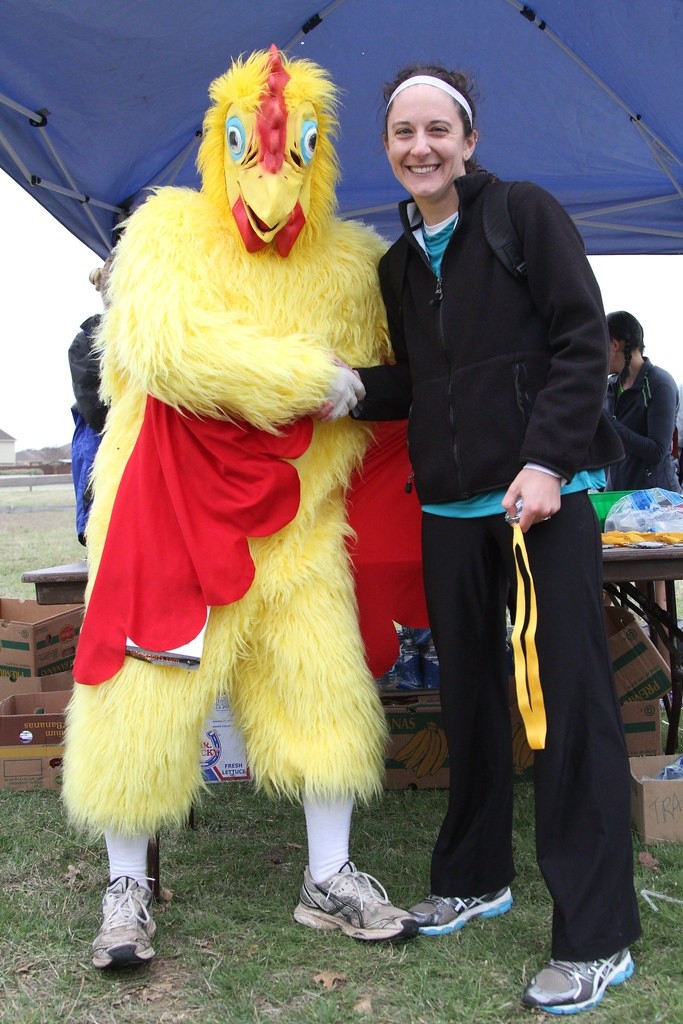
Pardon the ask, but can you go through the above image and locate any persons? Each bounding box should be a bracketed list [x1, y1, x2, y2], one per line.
[601, 310, 683, 706]
[322, 65, 646, 1018]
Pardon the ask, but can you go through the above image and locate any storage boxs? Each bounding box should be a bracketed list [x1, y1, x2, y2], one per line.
[628, 757, 683, 842]
[620, 699, 664, 761]
[377, 690, 452, 789]
[596, 607, 672, 702]
[197, 691, 253, 784]
[0, 594, 86, 794]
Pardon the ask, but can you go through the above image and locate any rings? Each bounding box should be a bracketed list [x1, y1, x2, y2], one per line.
[541, 515, 550, 520]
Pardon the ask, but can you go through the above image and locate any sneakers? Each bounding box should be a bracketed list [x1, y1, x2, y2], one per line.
[91, 876, 155, 969]
[407, 887, 513, 935]
[294, 863, 418, 941]
[522, 946, 634, 1015]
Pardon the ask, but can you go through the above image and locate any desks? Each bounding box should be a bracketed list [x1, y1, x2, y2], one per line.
[600, 542, 683, 754]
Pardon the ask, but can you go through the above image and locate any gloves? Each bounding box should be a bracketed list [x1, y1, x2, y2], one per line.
[320, 365, 366, 419]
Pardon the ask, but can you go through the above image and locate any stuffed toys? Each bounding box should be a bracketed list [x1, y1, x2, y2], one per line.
[54, 43, 422, 970]
